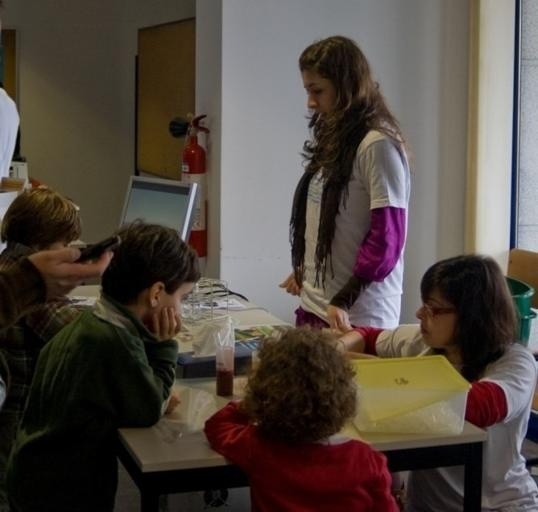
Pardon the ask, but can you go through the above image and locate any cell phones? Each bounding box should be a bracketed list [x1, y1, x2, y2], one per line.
[76, 236, 118, 263]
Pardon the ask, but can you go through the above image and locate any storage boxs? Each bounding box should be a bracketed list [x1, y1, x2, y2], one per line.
[351, 354, 473, 437]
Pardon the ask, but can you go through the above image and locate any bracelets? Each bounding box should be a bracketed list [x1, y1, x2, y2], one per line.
[339, 337, 350, 351]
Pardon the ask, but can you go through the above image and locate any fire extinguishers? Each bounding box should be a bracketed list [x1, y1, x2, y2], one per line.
[181, 114, 210, 257]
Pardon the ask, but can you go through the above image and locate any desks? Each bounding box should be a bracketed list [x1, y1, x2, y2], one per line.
[64, 285, 488, 511]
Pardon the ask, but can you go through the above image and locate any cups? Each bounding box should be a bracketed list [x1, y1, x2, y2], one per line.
[181, 281, 229, 325]
[215, 346, 233, 396]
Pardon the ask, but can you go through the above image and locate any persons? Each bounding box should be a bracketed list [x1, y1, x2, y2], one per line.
[1, 186, 79, 476]
[330, 253, 538, 512]
[0, 221, 204, 512]
[203, 324, 401, 511]
[269, 32, 412, 353]
[0, 242, 115, 322]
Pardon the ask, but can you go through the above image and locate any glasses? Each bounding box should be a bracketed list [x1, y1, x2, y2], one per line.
[423, 302, 455, 319]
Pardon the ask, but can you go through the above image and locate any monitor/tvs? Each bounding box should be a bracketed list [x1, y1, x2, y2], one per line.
[118, 175, 198, 245]
